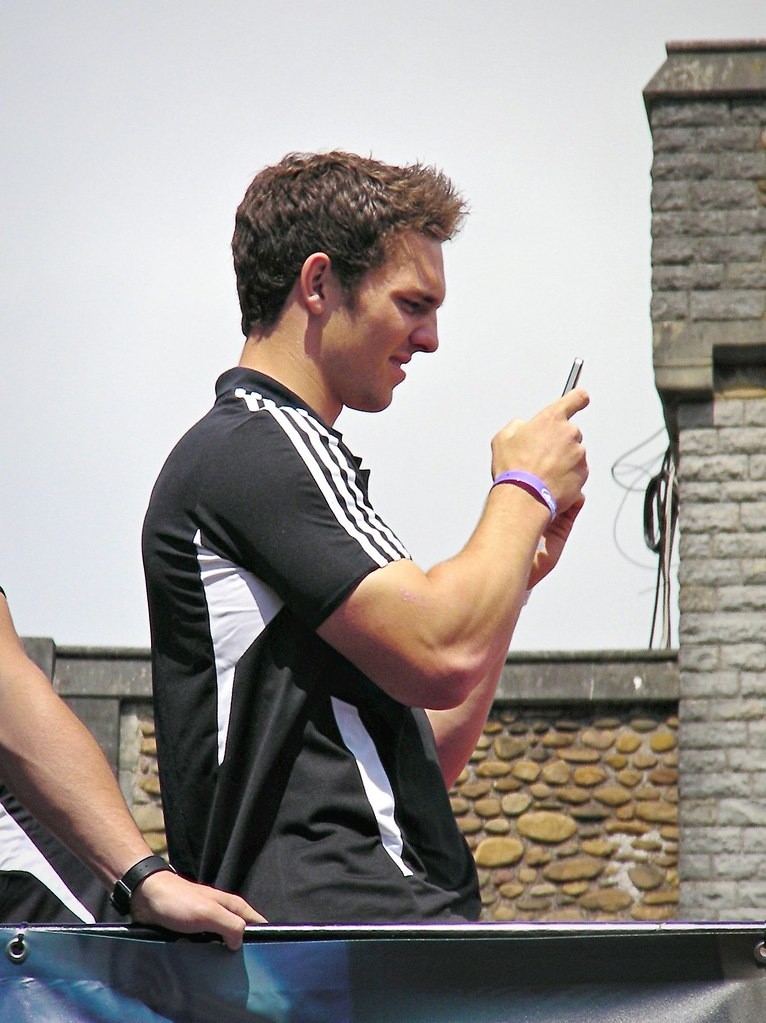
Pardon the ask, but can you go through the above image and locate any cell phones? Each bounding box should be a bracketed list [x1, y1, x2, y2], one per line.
[562, 359, 584, 396]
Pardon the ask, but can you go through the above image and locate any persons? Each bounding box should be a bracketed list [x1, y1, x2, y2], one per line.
[0, 587, 269, 950]
[140, 153, 590, 925]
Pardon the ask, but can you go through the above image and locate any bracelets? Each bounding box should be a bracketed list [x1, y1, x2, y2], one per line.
[109, 854, 176, 916]
[490, 471, 558, 528]
